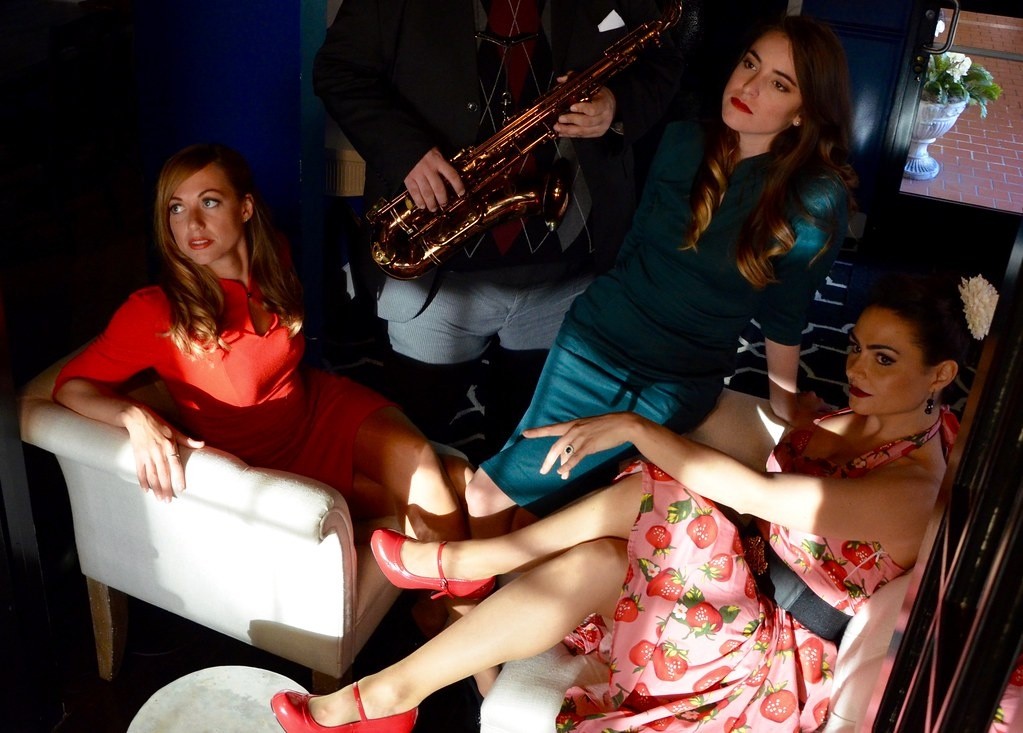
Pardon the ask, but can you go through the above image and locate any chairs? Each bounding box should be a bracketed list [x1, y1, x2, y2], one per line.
[19, 330, 468, 695]
[480, 387, 914, 733]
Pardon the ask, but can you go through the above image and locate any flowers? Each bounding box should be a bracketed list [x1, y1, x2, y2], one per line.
[955, 275, 1000, 340]
[924, 20, 1002, 121]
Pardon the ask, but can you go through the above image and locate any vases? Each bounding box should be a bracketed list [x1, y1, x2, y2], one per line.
[900, 93, 970, 180]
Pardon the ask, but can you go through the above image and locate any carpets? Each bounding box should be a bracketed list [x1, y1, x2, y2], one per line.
[125, 663, 309, 733]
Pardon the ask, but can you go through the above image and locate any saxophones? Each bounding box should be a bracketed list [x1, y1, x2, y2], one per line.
[348, 0, 686, 281]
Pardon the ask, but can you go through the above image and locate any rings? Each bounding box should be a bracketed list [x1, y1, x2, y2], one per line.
[168, 453, 180, 457]
[564, 446, 574, 455]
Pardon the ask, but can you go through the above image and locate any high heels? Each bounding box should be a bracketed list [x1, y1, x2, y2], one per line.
[371, 528, 495, 602]
[270, 682, 418, 733]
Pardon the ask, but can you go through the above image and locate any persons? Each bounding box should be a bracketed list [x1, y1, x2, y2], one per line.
[311, 0, 693, 462]
[51, 141, 502, 703]
[271, 275, 1000, 733]
[467, 16, 855, 593]
